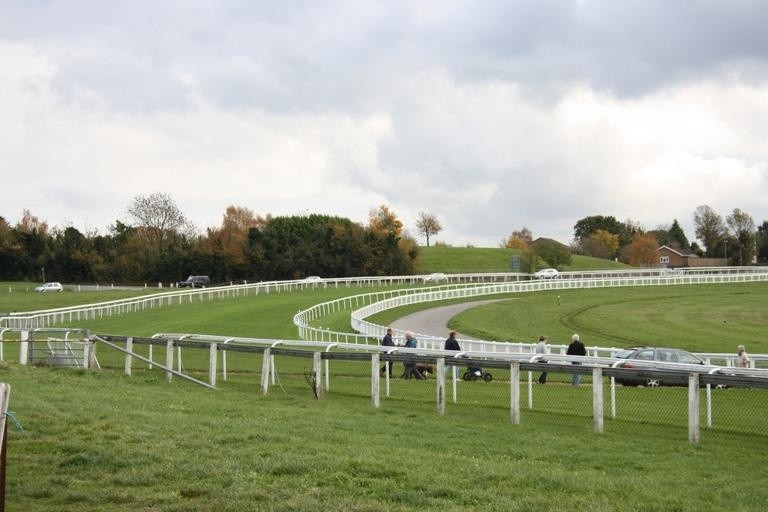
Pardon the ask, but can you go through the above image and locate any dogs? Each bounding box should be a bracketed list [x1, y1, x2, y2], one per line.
[399, 364, 435, 380]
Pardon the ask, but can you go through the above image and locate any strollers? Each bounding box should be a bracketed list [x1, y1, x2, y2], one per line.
[462, 356, 491, 382]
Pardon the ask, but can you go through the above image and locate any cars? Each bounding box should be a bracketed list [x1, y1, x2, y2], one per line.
[178, 278, 208, 288]
[608, 345, 733, 389]
[533, 268, 558, 279]
[34, 282, 63, 293]
[423, 273, 447, 283]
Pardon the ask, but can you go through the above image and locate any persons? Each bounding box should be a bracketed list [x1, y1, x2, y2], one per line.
[379, 330, 397, 379]
[404, 331, 416, 379]
[444, 331, 462, 381]
[737, 344, 750, 369]
[535, 335, 551, 383]
[566, 333, 590, 386]
